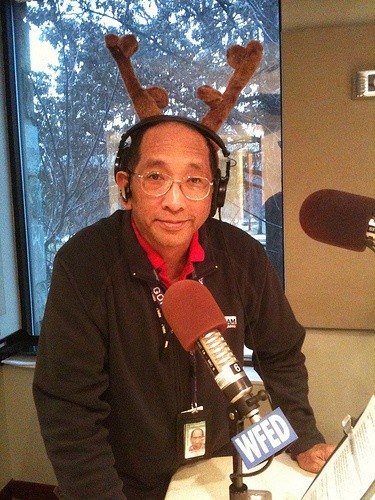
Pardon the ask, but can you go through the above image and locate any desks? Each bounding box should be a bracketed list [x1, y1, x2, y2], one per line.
[164, 452, 318, 500]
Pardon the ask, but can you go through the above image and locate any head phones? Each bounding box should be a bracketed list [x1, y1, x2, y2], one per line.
[114, 115, 231, 217]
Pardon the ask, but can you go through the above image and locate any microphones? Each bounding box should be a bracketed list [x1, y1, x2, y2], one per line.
[299, 189, 375, 252]
[162, 280, 267, 426]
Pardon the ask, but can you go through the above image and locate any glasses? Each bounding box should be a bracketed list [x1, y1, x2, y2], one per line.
[133, 171, 214, 201]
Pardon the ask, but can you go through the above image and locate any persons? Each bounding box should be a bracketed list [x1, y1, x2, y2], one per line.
[188, 429, 204, 451]
[31, 116, 340, 500]
